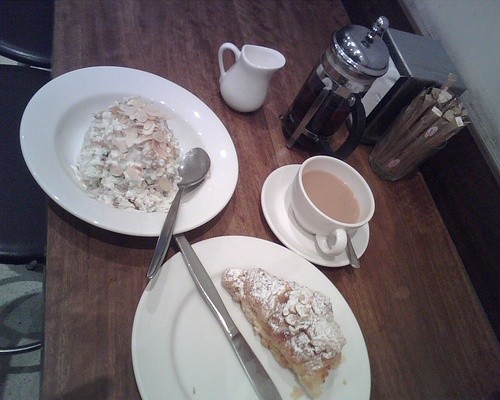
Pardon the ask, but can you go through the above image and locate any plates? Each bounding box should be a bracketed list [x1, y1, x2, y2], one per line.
[260, 164, 370, 268]
[131, 235, 371, 400]
[20, 66, 238, 237]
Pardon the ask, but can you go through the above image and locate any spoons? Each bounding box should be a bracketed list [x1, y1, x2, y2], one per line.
[147, 147, 210, 278]
[346, 233, 360, 268]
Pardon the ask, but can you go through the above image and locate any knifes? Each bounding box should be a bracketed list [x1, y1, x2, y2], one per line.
[174, 235, 282, 400]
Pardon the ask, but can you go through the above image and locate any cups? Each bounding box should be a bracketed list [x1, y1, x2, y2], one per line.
[369, 108, 446, 182]
[218, 42, 286, 113]
[292, 155, 375, 255]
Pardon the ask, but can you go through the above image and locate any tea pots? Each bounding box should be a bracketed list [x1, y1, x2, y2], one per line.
[283, 16, 391, 158]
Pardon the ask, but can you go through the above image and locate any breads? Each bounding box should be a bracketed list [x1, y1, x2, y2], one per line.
[220, 267, 347, 399]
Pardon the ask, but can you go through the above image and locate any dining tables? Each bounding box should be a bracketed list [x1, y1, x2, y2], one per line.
[40, 1, 500, 400]
